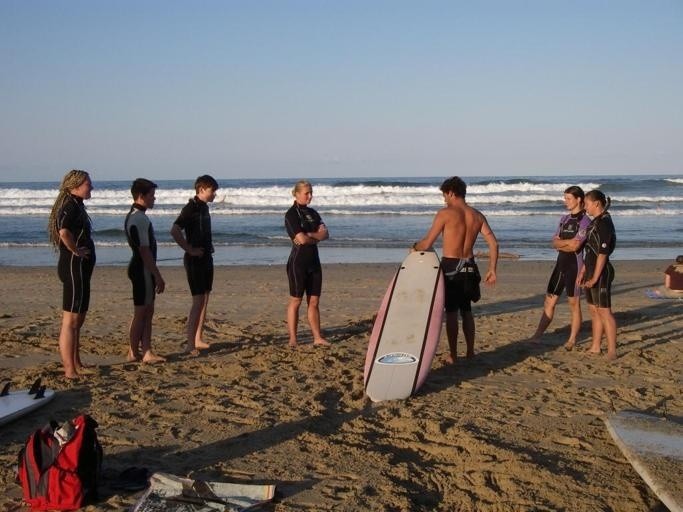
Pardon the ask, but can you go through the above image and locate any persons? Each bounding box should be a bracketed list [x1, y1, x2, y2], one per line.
[54, 169, 101, 381]
[666, 256, 683, 292]
[528, 186, 585, 350]
[411, 176, 499, 367]
[577, 192, 615, 362]
[127, 178, 166, 363]
[169, 176, 219, 355]
[286, 181, 333, 350]
